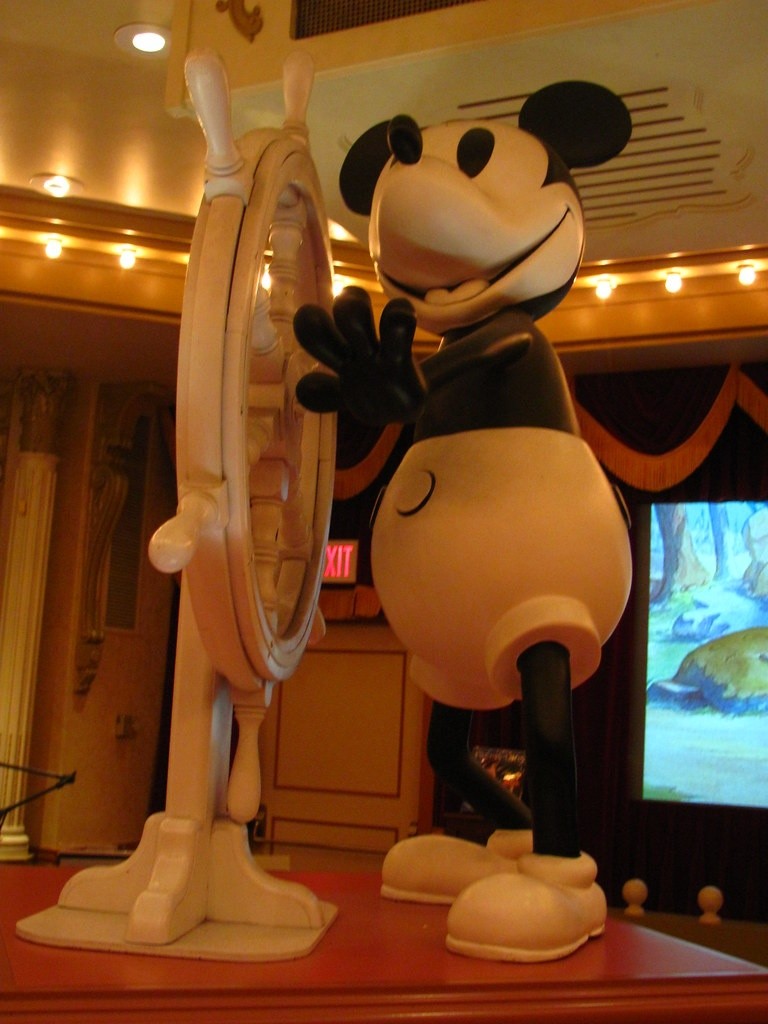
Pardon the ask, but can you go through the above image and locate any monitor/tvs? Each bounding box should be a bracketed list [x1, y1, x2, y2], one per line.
[628, 498, 768, 810]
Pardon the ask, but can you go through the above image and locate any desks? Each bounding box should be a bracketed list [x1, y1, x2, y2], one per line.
[0, 869, 768, 1024]
